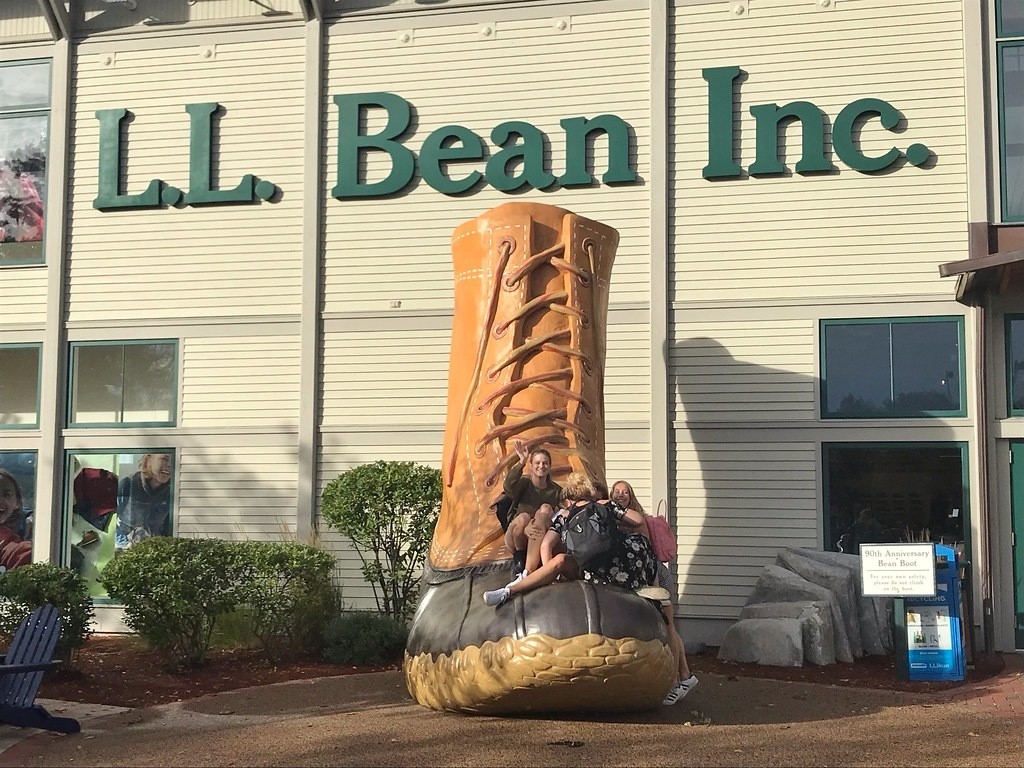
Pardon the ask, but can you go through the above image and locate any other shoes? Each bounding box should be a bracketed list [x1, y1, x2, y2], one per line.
[513, 548, 526, 572]
[76, 530, 97, 547]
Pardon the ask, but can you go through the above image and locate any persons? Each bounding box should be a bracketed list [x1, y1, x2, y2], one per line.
[0, 468, 34, 572]
[610, 481, 698, 705]
[483, 440, 669, 608]
[114, 454, 171, 547]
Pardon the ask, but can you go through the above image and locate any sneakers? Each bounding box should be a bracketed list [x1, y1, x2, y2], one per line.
[680, 675, 698, 698]
[505, 569, 527, 588]
[484, 588, 510, 606]
[663, 685, 684, 706]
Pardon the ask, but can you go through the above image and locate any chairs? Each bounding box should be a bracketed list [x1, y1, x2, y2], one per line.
[0, 604, 83, 735]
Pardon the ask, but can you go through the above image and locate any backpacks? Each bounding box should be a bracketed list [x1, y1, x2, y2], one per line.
[637, 498, 677, 563]
[490, 476, 530, 533]
[561, 501, 620, 573]
